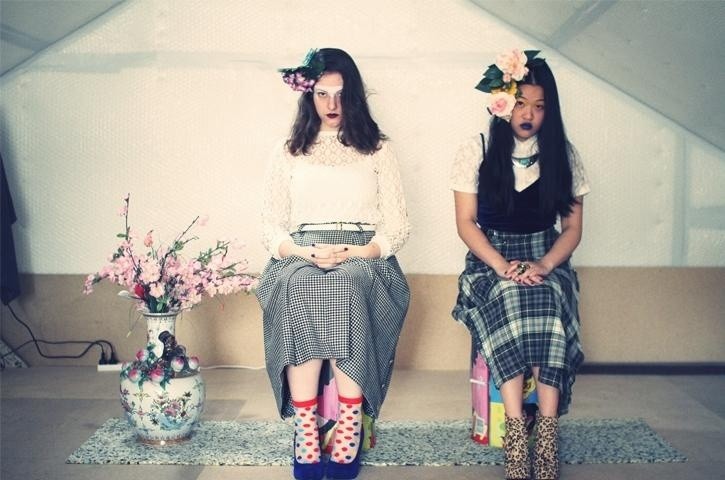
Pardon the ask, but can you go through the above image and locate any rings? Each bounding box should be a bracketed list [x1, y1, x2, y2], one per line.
[518, 263, 526, 272]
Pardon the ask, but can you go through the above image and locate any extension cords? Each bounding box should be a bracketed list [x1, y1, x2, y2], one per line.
[95, 359, 133, 373]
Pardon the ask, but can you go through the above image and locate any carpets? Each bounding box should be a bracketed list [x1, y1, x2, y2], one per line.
[68, 417, 687, 467]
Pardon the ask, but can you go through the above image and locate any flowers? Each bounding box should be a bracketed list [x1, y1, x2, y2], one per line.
[83, 193, 262, 337]
[475, 39, 541, 118]
[283, 65, 317, 92]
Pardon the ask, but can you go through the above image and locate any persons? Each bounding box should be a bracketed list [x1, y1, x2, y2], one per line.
[255, 49, 413, 478]
[450, 52, 591, 480]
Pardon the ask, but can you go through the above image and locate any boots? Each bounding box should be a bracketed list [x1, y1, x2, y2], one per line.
[504, 413, 559, 480]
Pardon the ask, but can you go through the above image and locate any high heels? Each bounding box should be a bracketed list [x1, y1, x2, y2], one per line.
[294, 424, 363, 480]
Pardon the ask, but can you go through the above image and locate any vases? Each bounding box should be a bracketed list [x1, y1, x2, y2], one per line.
[119, 309, 204, 444]
